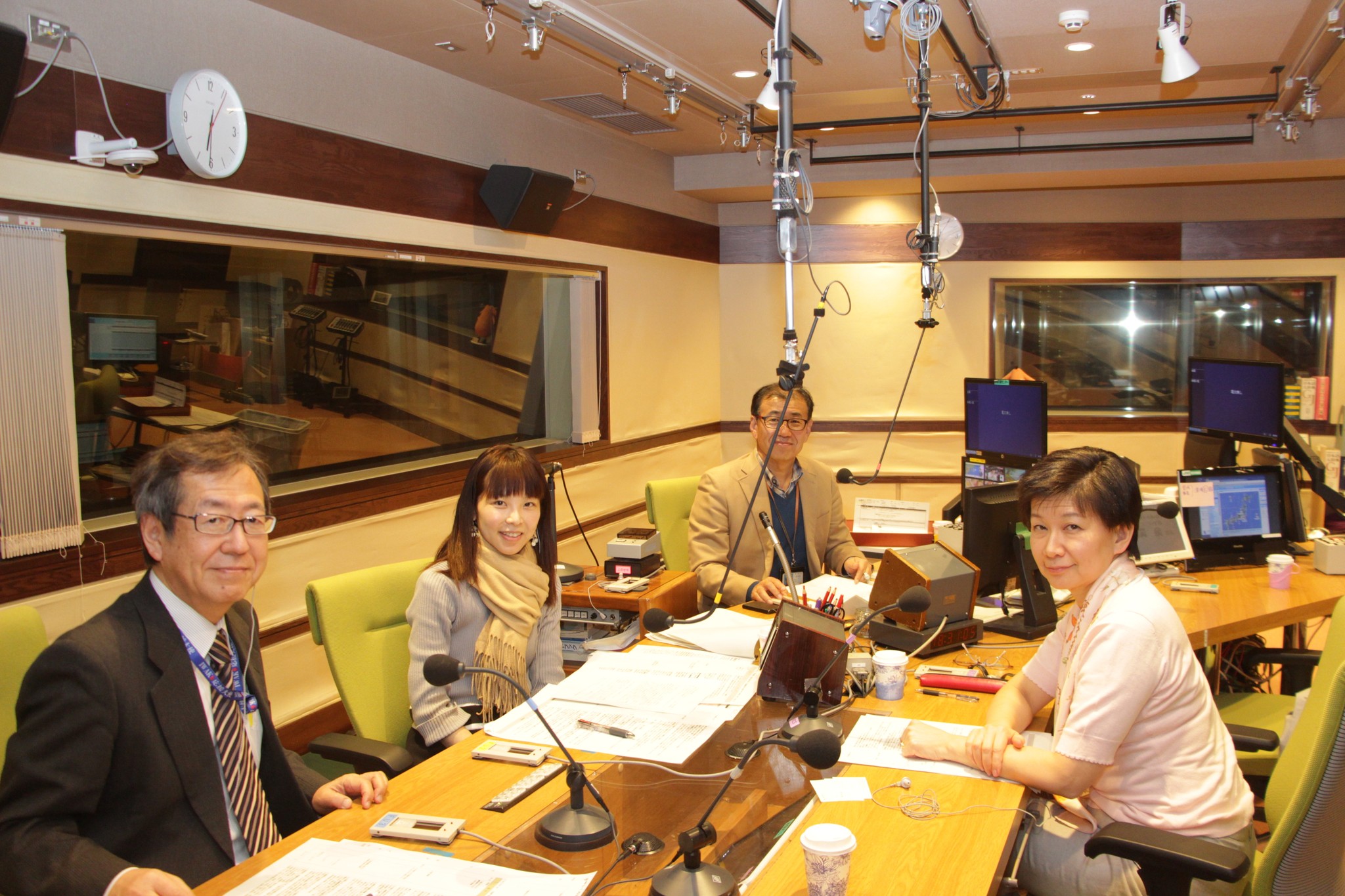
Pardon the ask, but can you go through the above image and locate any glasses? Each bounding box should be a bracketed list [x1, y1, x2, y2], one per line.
[754, 415, 808, 430]
[170, 511, 277, 535]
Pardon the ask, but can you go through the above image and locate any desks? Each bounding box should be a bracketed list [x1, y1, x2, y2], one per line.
[110, 404, 239, 445]
[191, 540, 1345, 896]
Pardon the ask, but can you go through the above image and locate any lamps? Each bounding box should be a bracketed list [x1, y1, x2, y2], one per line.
[734, 126, 751, 147]
[1156, 0, 1201, 83]
[1300, 92, 1321, 116]
[520, 25, 545, 51]
[756, 40, 779, 110]
[1275, 117, 1300, 142]
[864, 0, 894, 40]
[662, 89, 681, 115]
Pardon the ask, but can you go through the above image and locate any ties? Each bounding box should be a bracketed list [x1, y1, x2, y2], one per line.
[208, 630, 284, 858]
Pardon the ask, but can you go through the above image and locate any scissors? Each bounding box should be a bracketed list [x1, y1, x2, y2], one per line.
[821, 604, 846, 620]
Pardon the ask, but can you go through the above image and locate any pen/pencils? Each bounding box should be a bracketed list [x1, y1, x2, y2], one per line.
[577, 719, 635, 738]
[914, 688, 980, 703]
[802, 584, 843, 616]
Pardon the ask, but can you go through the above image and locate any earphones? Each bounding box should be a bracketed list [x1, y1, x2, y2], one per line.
[892, 776, 911, 789]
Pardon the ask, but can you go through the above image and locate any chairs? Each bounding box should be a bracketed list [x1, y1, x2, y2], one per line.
[644, 475, 701, 573]
[1084, 594, 1345, 896]
[75, 365, 119, 424]
[306, 557, 434, 781]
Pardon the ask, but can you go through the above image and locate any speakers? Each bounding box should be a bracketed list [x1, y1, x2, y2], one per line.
[478, 164, 574, 235]
[1183, 433, 1236, 468]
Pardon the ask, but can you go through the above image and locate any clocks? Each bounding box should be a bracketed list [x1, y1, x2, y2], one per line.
[915, 211, 964, 260]
[169, 69, 248, 180]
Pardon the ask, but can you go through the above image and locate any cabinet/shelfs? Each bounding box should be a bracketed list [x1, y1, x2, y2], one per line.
[241, 328, 298, 387]
[559, 564, 697, 677]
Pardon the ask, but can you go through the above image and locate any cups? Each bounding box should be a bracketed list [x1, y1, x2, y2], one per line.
[1265, 553, 1301, 590]
[799, 822, 858, 896]
[872, 650, 910, 701]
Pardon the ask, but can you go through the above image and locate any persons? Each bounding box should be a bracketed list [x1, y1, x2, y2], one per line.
[403, 443, 565, 755]
[688, 382, 872, 611]
[1, 435, 389, 896]
[899, 447, 1257, 896]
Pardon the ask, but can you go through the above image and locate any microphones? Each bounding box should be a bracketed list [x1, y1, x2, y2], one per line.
[786, 584, 932, 722]
[643, 317, 826, 633]
[671, 729, 841, 864]
[423, 654, 612, 815]
[1140, 501, 1180, 520]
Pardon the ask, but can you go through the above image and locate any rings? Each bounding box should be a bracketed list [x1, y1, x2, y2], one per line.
[899, 738, 904, 746]
[863, 572, 870, 580]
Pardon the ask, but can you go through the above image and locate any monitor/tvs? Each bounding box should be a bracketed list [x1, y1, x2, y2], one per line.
[1177, 464, 1291, 543]
[960, 456, 1037, 514]
[1127, 499, 1198, 565]
[963, 478, 1038, 598]
[84, 312, 160, 364]
[1187, 356, 1285, 448]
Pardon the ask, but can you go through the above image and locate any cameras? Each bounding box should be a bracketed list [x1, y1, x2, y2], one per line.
[963, 377, 1049, 460]
